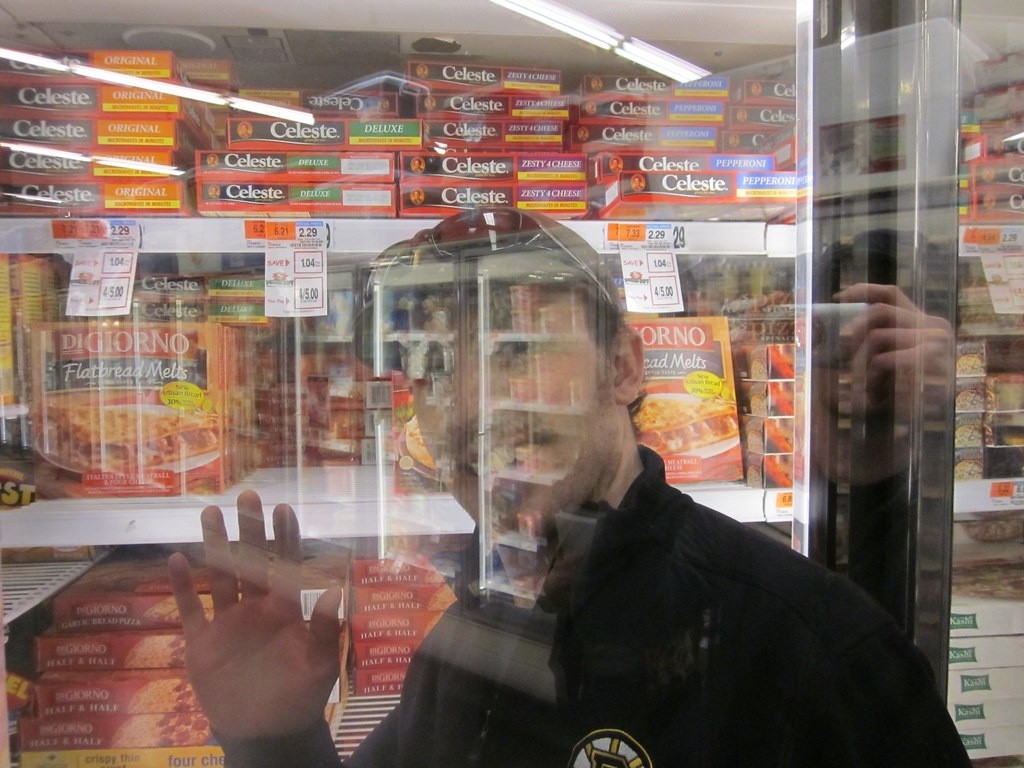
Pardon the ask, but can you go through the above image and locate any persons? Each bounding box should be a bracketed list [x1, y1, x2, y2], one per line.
[167, 209, 976, 768]
[751, 227, 958, 643]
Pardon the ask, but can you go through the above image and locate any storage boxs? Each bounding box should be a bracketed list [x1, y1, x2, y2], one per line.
[0, 41, 1024, 223]
[10, 556, 1024, 768]
[0, 253, 1024, 499]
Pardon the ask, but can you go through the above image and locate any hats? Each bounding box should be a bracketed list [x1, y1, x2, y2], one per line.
[372, 210, 608, 285]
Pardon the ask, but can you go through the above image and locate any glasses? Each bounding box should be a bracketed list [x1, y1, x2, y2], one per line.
[364, 206, 626, 324]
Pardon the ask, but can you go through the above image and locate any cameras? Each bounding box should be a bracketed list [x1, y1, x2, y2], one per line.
[763, 303, 872, 372]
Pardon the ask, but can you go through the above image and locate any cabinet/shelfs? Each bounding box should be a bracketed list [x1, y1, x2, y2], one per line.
[0, 0, 1024, 768]
